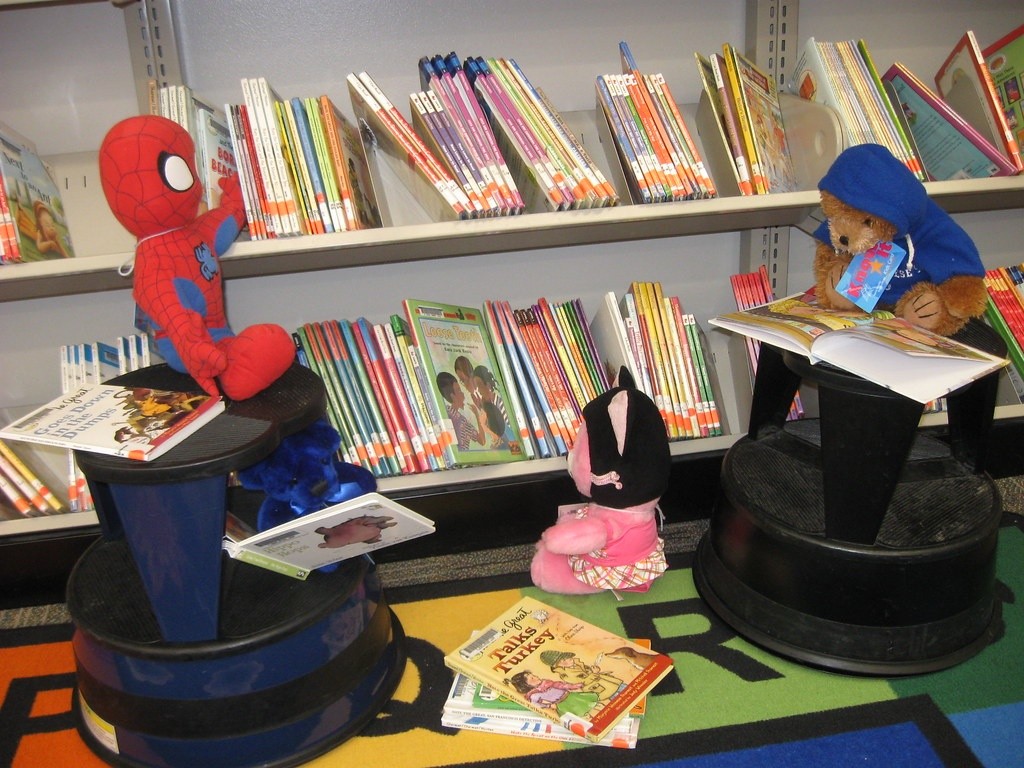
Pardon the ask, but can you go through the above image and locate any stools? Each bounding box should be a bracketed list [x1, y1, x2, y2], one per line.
[63, 358, 404, 768]
[690, 324, 1007, 676]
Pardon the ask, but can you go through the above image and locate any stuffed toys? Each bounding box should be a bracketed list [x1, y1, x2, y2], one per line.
[531, 366, 673, 594]
[238, 421, 378, 572]
[99, 113, 295, 401]
[811, 143, 987, 335]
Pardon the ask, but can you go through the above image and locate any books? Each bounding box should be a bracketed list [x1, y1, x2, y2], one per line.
[0, 333, 165, 518]
[0, 135, 75, 264]
[294, 298, 610, 476]
[695, 43, 797, 195]
[596, 41, 716, 206]
[441, 596, 673, 749]
[787, 22, 1023, 179]
[221, 492, 435, 580]
[160, 53, 621, 241]
[708, 287, 1011, 404]
[983, 262, 1024, 398]
[0, 383, 226, 462]
[620, 280, 731, 442]
[923, 398, 947, 414]
[730, 265, 805, 421]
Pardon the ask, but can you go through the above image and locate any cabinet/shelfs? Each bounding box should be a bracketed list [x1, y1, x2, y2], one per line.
[0, 0, 1024, 549]
